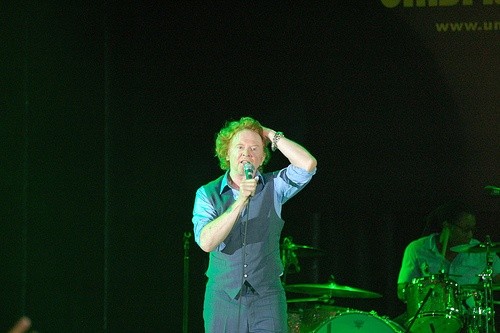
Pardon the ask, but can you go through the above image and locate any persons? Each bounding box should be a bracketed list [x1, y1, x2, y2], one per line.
[192, 117, 318, 333]
[393, 197, 500, 325]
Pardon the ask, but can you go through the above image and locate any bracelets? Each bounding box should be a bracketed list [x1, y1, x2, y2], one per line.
[271, 131, 285, 152]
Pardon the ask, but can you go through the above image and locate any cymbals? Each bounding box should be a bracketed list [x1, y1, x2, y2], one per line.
[461, 283, 500, 290]
[279, 244, 319, 250]
[280, 284, 384, 299]
[449, 243, 500, 253]
[423, 273, 464, 277]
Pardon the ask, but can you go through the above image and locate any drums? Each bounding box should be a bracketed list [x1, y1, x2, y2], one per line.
[463, 306, 495, 333]
[459, 291, 495, 333]
[403, 275, 464, 333]
[288, 301, 407, 333]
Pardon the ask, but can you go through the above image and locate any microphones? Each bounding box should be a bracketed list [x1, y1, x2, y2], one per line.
[244, 162, 255, 197]
